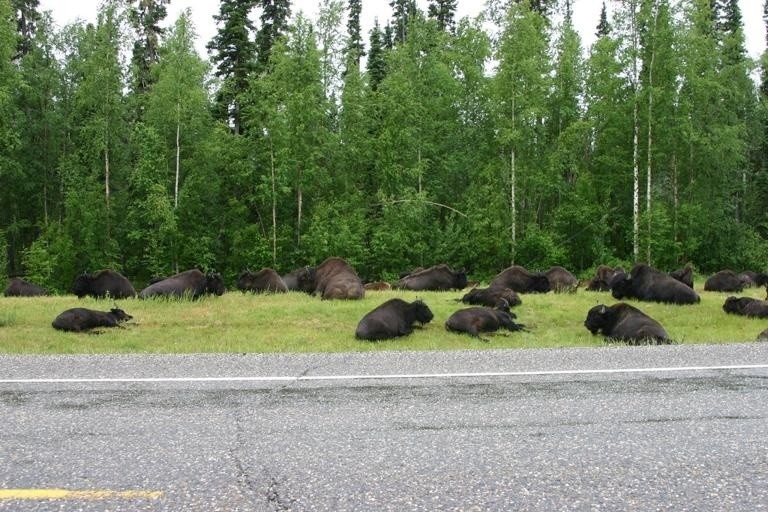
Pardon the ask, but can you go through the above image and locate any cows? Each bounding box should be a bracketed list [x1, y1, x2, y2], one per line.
[611, 264, 701, 305]
[234, 256, 469, 301]
[355, 297, 434, 340]
[4, 269, 224, 301]
[444, 298, 526, 342]
[488, 266, 552, 294]
[723, 296, 768, 319]
[52, 303, 133, 336]
[539, 263, 768, 293]
[584, 302, 671, 345]
